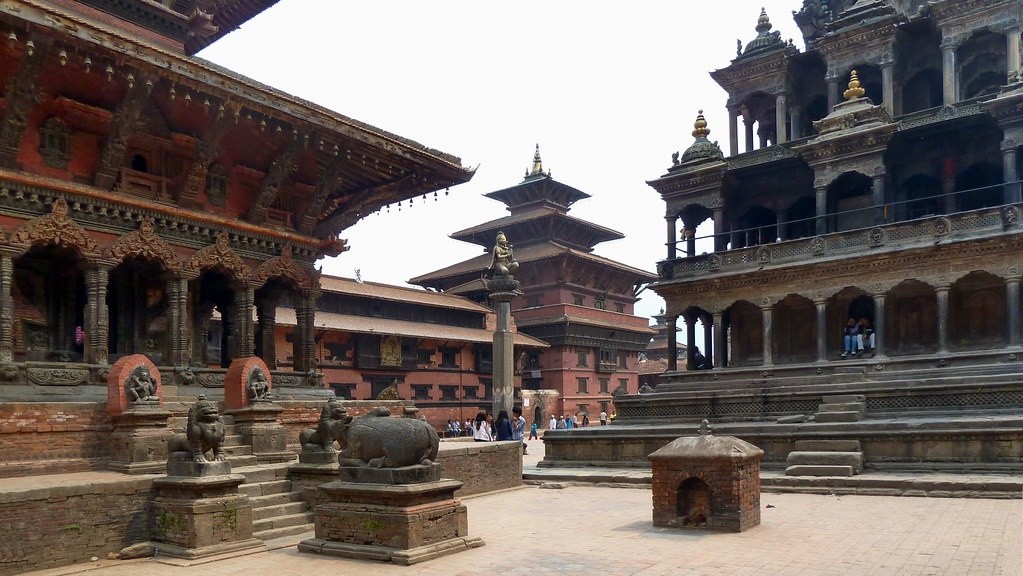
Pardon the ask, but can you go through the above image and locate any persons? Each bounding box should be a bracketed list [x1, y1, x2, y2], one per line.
[487, 231, 519, 276]
[691, 345, 713, 371]
[855, 315, 876, 356]
[841, 317, 857, 357]
[441, 406, 617, 456]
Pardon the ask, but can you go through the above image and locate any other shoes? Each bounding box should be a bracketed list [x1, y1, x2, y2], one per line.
[841, 350, 877, 356]
